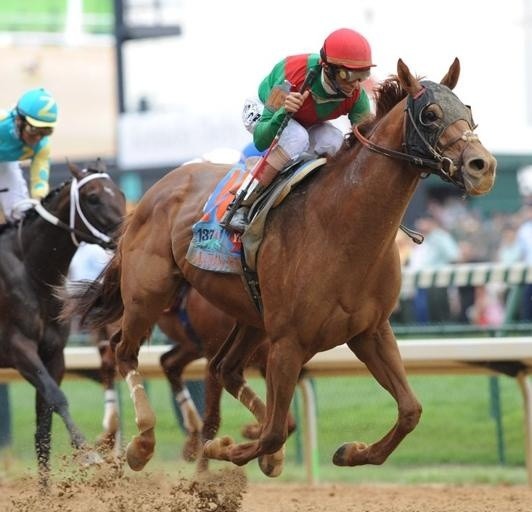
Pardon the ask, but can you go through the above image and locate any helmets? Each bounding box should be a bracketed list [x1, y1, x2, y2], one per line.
[319, 28, 377, 69]
[13, 89, 58, 127]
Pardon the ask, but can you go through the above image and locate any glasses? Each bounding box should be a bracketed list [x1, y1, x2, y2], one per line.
[321, 48, 370, 82]
[16, 106, 53, 136]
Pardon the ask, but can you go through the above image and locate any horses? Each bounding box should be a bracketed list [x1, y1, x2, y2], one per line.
[0, 156, 127, 488]
[94, 279, 308, 474]
[43, 56, 498, 479]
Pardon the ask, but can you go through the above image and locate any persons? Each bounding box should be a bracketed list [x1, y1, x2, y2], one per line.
[0, 86, 60, 232]
[216, 27, 374, 231]
[68, 243, 167, 344]
[392, 198, 532, 336]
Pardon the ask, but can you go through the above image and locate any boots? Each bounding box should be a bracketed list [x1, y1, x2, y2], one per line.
[221, 174, 266, 231]
